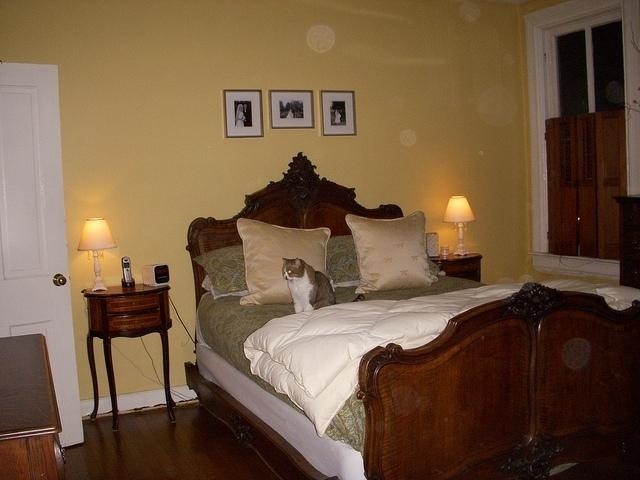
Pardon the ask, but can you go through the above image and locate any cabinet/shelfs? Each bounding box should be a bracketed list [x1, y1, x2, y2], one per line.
[612, 195, 640, 293]
[1, 334, 65, 480]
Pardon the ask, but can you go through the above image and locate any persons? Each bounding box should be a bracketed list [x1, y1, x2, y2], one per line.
[334, 108, 345, 125]
[234, 102, 254, 128]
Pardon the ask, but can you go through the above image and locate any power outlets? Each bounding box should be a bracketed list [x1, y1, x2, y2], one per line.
[153, 265, 171, 285]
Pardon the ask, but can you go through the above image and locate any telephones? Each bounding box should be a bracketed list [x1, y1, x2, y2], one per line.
[121, 256, 135, 288]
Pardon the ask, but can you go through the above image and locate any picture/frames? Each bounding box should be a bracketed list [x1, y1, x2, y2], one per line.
[221, 88, 357, 139]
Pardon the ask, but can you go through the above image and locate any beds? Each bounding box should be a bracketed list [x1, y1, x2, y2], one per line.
[183, 154, 640, 480]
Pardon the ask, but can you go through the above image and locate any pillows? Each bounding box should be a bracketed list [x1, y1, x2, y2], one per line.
[192, 209, 443, 306]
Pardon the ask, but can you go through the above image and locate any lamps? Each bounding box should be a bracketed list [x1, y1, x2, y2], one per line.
[77, 217, 118, 293]
[442, 193, 477, 256]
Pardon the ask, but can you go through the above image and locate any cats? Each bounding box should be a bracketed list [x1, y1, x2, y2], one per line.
[281, 257, 365, 313]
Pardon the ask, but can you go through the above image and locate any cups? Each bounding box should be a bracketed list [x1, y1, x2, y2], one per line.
[440, 247, 449, 260]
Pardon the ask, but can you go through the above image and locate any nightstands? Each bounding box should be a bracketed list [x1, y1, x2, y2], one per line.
[80, 285, 178, 432]
[429, 253, 483, 282]
